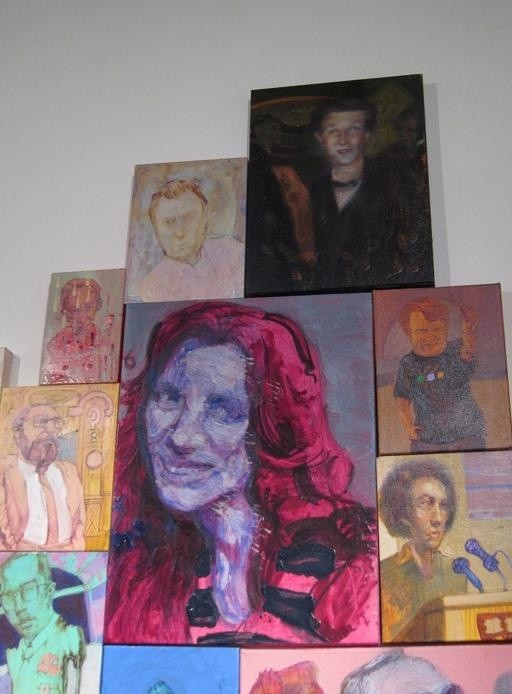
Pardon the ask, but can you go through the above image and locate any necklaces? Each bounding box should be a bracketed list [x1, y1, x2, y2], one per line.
[327, 175, 362, 187]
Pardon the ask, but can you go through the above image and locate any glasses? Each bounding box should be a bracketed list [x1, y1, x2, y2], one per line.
[20, 414, 64, 430]
[0, 578, 51, 613]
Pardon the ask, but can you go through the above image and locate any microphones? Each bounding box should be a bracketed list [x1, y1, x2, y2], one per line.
[451, 557, 485, 596]
[465, 539, 500, 571]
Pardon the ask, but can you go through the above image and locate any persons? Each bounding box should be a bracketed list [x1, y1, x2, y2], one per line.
[385, 297, 490, 450]
[263, 91, 402, 293]
[0, 549, 88, 694]
[42, 274, 120, 384]
[128, 174, 243, 303]
[95, 297, 380, 651]
[376, 460, 470, 645]
[1, 400, 88, 549]
